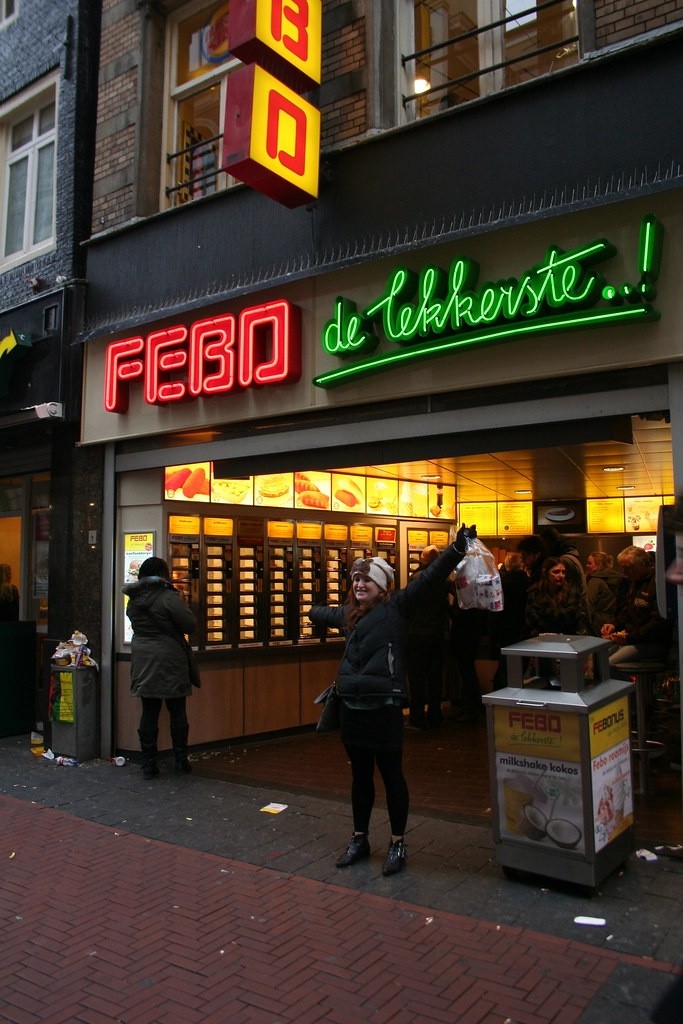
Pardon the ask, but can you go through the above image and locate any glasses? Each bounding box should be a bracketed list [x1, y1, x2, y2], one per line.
[519, 551, 532, 559]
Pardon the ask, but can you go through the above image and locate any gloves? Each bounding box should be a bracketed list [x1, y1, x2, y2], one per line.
[455, 524, 477, 553]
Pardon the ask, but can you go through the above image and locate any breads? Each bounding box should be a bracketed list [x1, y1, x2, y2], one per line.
[336, 490, 356, 507]
[295, 481, 319, 493]
[296, 473, 310, 481]
[300, 492, 328, 508]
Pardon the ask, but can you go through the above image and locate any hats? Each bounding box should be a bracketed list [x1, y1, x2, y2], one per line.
[421, 545, 439, 565]
[350, 557, 394, 592]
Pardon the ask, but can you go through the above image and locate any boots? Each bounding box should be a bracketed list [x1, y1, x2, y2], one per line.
[169, 724, 191, 776]
[137, 728, 159, 780]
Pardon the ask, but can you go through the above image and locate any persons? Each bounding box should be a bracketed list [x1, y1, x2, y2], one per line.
[121, 557, 201, 781]
[403, 527, 658, 736]
[0, 563, 19, 623]
[312, 522, 477, 875]
[649, 486, 683, 1024]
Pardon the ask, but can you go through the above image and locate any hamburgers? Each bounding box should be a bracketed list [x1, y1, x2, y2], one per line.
[130, 560, 143, 579]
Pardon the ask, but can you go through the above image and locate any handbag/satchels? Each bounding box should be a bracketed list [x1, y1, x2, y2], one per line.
[313, 681, 340, 732]
[455, 535, 504, 611]
[185, 643, 201, 689]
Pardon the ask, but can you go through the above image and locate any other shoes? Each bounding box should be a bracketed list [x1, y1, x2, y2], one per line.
[404, 721, 422, 731]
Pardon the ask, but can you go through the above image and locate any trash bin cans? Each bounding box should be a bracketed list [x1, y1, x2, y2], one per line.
[480, 633, 636, 897]
[49, 662, 102, 760]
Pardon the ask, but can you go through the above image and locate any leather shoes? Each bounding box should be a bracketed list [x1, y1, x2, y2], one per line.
[336, 832, 370, 868]
[383, 836, 407, 876]
